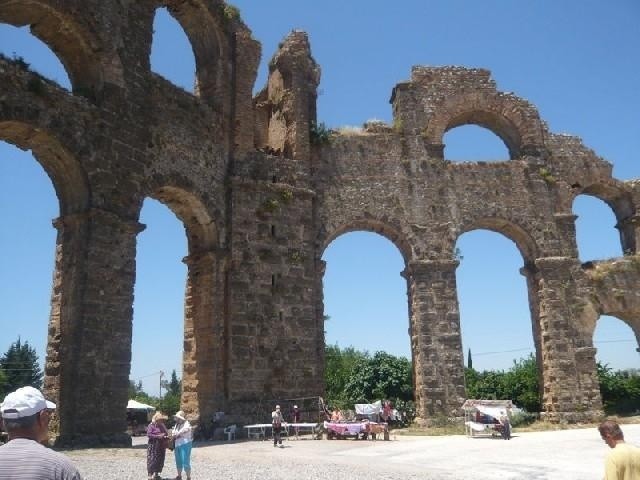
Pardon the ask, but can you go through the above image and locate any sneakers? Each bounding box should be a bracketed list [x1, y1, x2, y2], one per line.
[176, 476, 182, 479]
[149, 475, 161, 479]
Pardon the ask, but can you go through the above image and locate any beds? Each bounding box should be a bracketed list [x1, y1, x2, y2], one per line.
[465, 421, 512, 438]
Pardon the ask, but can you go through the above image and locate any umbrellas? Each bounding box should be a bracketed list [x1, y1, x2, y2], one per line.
[126, 398, 156, 411]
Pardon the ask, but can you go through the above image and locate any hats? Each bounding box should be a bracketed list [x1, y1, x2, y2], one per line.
[172, 411, 186, 421]
[276, 405, 280, 408]
[294, 405, 298, 408]
[1, 385, 57, 419]
[152, 413, 169, 422]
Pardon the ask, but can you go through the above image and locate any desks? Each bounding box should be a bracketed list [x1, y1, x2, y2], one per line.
[244, 423, 321, 441]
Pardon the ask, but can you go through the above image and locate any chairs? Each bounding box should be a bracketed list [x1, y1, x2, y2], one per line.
[224, 424, 237, 441]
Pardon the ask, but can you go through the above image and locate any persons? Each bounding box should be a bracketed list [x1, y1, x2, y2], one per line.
[293, 404, 301, 440]
[271, 404, 287, 447]
[500, 411, 511, 440]
[147, 411, 171, 480]
[331, 406, 343, 423]
[170, 411, 192, 480]
[0, 385, 82, 480]
[598, 421, 640, 480]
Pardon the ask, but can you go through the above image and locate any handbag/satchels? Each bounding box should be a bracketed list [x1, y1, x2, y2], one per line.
[168, 439, 174, 449]
